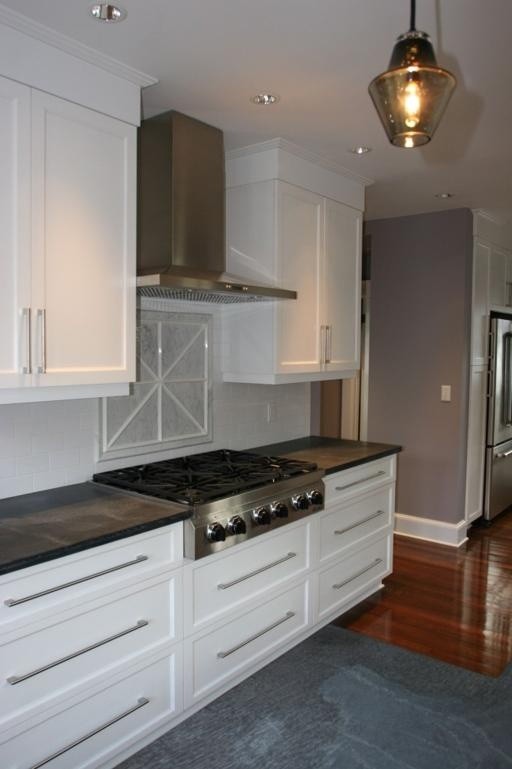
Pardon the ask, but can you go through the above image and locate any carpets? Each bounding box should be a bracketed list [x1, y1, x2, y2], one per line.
[114, 622, 510, 768]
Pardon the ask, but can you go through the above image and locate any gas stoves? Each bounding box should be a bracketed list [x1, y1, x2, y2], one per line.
[92, 448, 325, 560]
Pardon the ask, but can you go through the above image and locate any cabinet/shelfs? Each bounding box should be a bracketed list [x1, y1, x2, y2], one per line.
[219, 177, 365, 384]
[0, 523, 182, 768]
[313, 452, 399, 631]
[1, 73, 139, 405]
[182, 517, 312, 720]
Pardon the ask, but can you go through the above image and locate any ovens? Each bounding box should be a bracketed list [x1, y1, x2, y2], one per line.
[485, 314, 511, 528]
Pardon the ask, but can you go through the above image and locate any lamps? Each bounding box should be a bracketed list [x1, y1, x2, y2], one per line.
[368, 0, 456, 151]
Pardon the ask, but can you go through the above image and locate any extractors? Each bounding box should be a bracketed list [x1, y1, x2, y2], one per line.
[137, 109, 300, 317]
